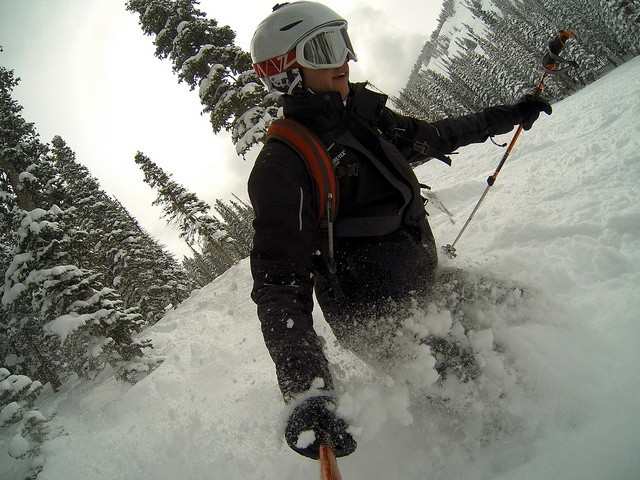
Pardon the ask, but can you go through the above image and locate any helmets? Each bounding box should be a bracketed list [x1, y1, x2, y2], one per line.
[250, 0, 348, 95]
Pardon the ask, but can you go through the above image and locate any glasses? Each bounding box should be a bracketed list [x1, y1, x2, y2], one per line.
[253, 22, 358, 78]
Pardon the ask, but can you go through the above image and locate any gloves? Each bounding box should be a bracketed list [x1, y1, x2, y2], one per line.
[284, 391, 357, 460]
[508, 94, 552, 131]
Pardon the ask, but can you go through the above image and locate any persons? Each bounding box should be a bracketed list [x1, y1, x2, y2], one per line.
[247, 0, 552, 460]
